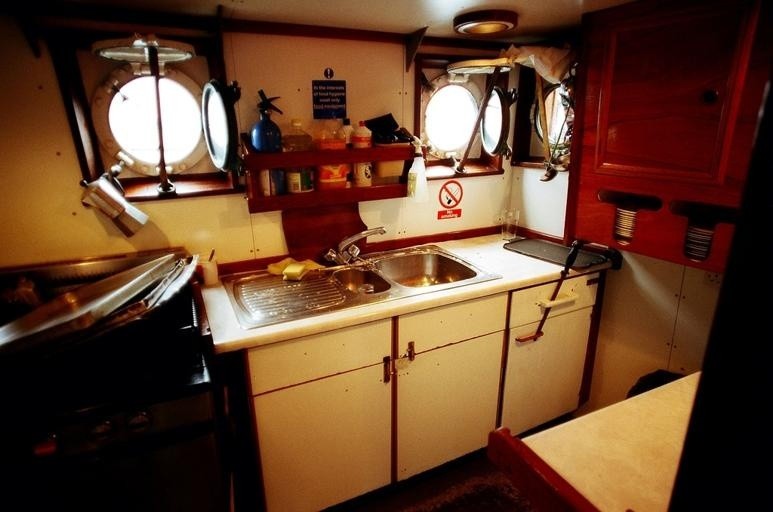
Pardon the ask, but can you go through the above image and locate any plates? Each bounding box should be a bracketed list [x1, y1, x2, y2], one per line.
[611, 207, 713, 260]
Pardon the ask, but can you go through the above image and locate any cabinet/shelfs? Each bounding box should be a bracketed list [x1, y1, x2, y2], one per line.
[238, 129, 417, 214]
[242, 315, 396, 511]
[396, 291, 511, 485]
[500, 270, 601, 436]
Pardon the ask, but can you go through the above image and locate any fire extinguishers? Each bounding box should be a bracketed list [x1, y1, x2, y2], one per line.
[251, 89, 286, 197]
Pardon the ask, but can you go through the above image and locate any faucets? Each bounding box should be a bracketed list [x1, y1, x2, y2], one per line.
[338, 226, 386, 265]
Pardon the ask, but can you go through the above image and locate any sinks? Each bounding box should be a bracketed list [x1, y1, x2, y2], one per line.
[331, 265, 393, 296]
[373, 250, 479, 292]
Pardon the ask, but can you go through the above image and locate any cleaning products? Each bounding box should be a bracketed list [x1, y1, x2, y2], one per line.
[406, 135, 428, 202]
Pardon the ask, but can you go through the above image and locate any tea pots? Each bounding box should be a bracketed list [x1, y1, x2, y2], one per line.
[79, 170, 149, 237]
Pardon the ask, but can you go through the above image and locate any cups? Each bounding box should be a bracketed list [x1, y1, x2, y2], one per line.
[500, 207, 520, 240]
[197, 260, 219, 286]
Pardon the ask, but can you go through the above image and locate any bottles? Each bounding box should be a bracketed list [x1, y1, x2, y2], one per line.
[342, 118, 375, 185]
[250, 109, 281, 197]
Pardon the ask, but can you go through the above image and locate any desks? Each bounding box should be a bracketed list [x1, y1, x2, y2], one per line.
[43, 341, 233, 512]
[486, 370, 702, 511]
